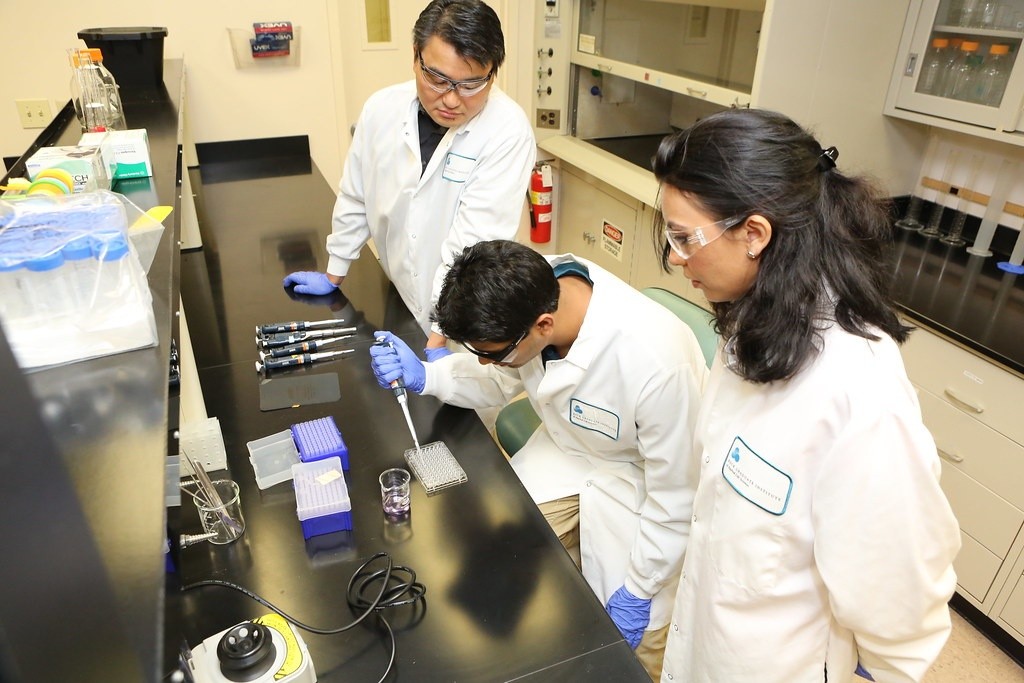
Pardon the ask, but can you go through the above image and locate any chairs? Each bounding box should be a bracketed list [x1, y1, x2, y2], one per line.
[496, 287, 722, 467]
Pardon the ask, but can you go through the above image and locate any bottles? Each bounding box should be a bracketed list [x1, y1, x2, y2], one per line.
[915, 38, 1013, 106]
[69, 49, 127, 132]
[0, 210, 152, 370]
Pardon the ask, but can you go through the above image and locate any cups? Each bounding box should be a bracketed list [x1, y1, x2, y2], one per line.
[207, 533, 253, 580]
[383, 511, 412, 544]
[193, 479, 246, 545]
[379, 468, 411, 515]
[958, 1, 1023, 31]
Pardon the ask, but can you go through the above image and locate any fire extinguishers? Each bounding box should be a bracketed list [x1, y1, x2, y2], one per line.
[526, 159, 557, 244]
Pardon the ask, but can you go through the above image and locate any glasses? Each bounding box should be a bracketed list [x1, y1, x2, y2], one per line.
[457, 328, 530, 364]
[663, 206, 777, 260]
[416, 49, 494, 97]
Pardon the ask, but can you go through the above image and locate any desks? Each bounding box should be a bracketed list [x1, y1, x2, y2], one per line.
[162, 154, 655, 683]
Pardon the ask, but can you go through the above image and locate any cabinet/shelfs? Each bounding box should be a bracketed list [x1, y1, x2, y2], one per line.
[529, 0, 933, 215]
[883, 0, 1024, 146]
[556, 160, 721, 318]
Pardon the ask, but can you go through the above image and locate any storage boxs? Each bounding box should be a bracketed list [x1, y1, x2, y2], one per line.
[78, 128, 153, 177]
[293, 474, 354, 541]
[0, 192, 162, 375]
[25, 146, 112, 196]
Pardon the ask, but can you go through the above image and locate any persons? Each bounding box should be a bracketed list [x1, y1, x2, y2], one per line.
[282, 0, 537, 363]
[370, 240, 711, 683]
[658, 109, 961, 683]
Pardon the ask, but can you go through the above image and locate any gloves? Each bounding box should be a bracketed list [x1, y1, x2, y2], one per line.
[424, 345, 454, 363]
[370, 331, 426, 394]
[602, 585, 651, 653]
[282, 271, 338, 296]
